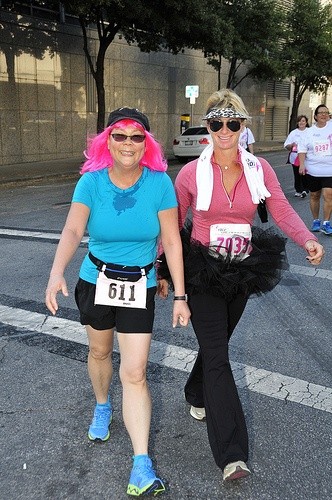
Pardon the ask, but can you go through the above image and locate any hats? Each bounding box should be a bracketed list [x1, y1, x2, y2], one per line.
[202, 107, 253, 120]
[107, 109, 150, 134]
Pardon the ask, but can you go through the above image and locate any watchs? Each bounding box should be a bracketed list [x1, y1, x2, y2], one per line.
[174, 294, 188, 302]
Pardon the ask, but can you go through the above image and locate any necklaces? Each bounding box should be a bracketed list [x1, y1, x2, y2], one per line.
[221, 163, 234, 170]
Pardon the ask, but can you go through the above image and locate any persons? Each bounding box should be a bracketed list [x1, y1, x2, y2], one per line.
[297, 105, 332, 234]
[239, 127, 256, 155]
[157, 88, 325, 480]
[45, 105, 191, 496]
[284, 115, 310, 198]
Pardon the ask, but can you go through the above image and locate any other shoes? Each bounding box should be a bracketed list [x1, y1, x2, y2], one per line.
[295, 192, 300, 196]
[301, 191, 307, 198]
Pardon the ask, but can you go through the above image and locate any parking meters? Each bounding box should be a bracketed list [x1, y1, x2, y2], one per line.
[180, 113, 190, 135]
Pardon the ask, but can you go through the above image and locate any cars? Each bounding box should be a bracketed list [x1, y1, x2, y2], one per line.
[173, 125, 213, 163]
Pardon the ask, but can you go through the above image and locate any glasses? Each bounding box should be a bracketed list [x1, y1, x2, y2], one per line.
[209, 121, 242, 132]
[110, 134, 145, 143]
[317, 111, 329, 115]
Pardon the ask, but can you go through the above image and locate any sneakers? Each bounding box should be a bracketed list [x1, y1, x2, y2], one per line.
[189, 404, 207, 422]
[127, 465, 166, 496]
[320, 222, 332, 235]
[311, 220, 321, 232]
[88, 396, 113, 442]
[223, 460, 250, 482]
